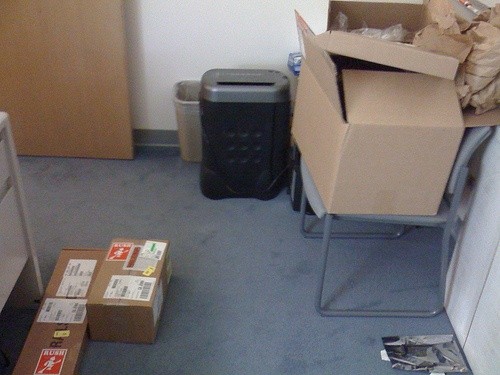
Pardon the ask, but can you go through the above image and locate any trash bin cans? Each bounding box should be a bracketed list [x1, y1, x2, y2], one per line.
[173, 79, 204, 163]
[197, 69, 291, 200]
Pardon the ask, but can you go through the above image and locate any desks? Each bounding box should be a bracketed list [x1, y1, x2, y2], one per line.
[0, 110, 43, 312]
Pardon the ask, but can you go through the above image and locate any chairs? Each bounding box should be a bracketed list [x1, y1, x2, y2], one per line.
[300, 124, 495, 317]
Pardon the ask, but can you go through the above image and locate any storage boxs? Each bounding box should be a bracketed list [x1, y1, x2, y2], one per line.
[290, 0, 500, 215]
[12, 237, 176, 375]
